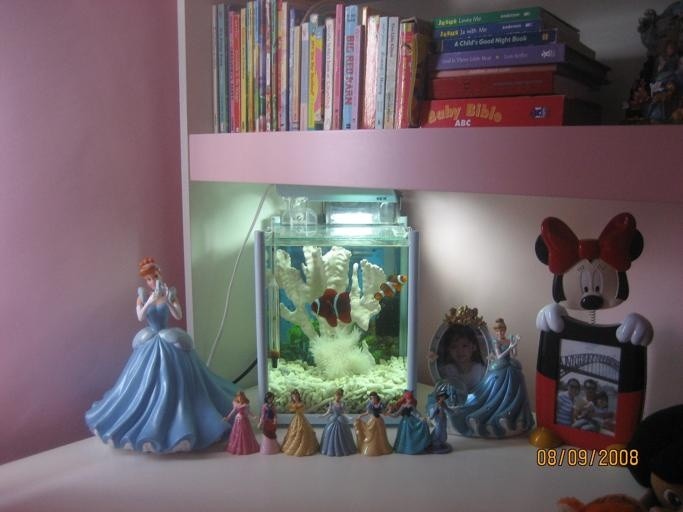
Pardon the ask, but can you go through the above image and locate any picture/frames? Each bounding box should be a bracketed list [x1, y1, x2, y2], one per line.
[426, 302, 495, 393]
[529, 308, 654, 466]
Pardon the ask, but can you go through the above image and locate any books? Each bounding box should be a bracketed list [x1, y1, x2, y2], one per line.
[206, 1, 609, 131]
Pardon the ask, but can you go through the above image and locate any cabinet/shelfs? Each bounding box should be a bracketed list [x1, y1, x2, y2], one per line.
[0, 1, 683, 511]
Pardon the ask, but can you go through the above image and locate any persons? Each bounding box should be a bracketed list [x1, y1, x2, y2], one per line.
[315, 388, 358, 457]
[255, 391, 280, 454]
[423, 391, 459, 453]
[84, 257, 248, 456]
[439, 317, 534, 439]
[557, 378, 616, 430]
[352, 391, 395, 457]
[222, 390, 260, 455]
[442, 326, 488, 390]
[382, 390, 431, 454]
[279, 388, 319, 456]
